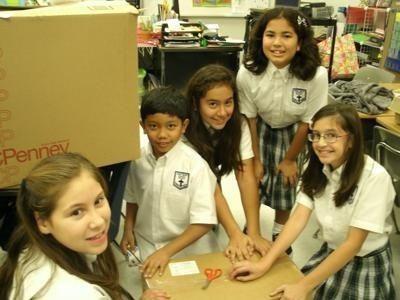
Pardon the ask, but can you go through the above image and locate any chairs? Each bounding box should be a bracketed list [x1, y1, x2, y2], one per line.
[351, 64, 396, 83]
[371, 125, 400, 234]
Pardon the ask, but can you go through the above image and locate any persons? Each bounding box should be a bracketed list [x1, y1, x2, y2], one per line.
[228, 103, 396, 300]
[1, 152, 166, 299]
[183, 64, 273, 261]
[234, 6, 329, 262]
[120, 86, 219, 300]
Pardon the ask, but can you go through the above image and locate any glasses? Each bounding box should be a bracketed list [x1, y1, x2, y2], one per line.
[307, 133, 350, 142]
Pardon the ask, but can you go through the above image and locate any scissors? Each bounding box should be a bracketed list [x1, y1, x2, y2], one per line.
[202, 269, 222, 290]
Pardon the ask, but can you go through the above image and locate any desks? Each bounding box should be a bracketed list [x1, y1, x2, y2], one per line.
[356, 83, 400, 136]
[155, 42, 243, 86]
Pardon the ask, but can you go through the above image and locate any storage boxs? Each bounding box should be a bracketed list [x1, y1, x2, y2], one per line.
[140, 241, 314, 300]
[0, 0, 142, 189]
